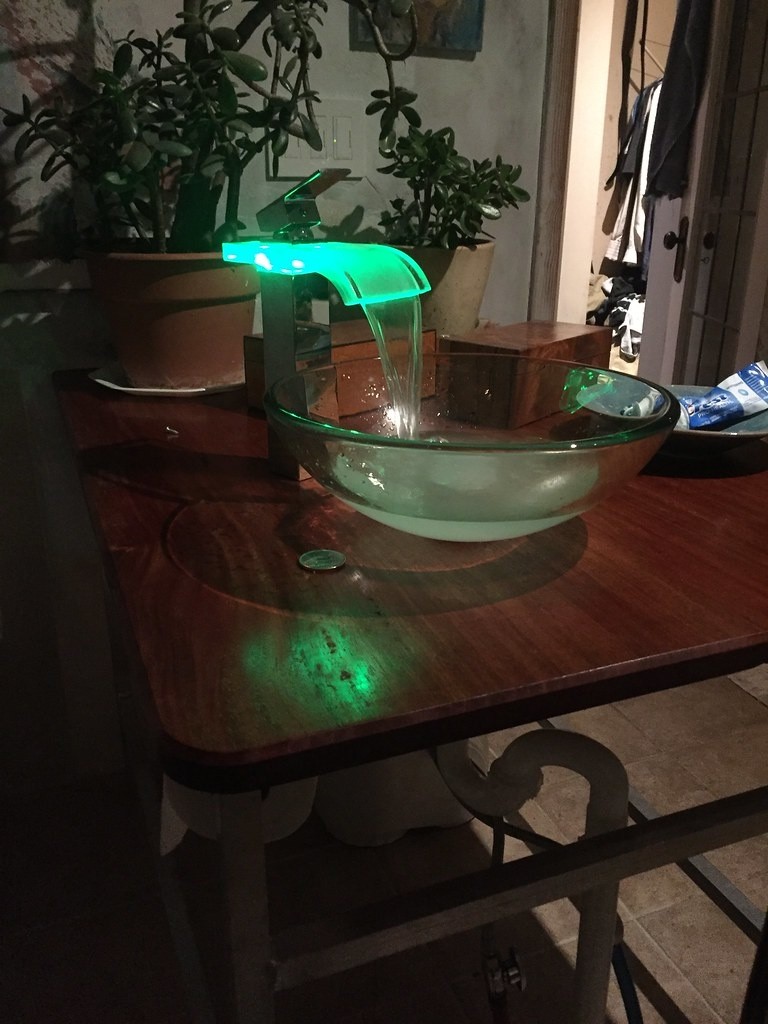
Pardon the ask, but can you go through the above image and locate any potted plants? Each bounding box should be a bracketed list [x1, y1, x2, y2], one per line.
[0, 0, 528, 390]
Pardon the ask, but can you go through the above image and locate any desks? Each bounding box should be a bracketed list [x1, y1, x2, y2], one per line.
[51, 367, 768, 1024]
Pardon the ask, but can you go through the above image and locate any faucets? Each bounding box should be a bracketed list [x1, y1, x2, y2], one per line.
[222, 167, 432, 482]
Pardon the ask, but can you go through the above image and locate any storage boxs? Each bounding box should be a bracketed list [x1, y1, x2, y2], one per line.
[437, 321, 613, 431]
[244, 319, 437, 417]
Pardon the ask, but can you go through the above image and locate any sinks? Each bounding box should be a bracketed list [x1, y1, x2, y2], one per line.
[263, 352, 681, 542]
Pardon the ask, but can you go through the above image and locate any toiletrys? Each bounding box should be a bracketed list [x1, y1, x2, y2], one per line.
[674, 360, 768, 430]
